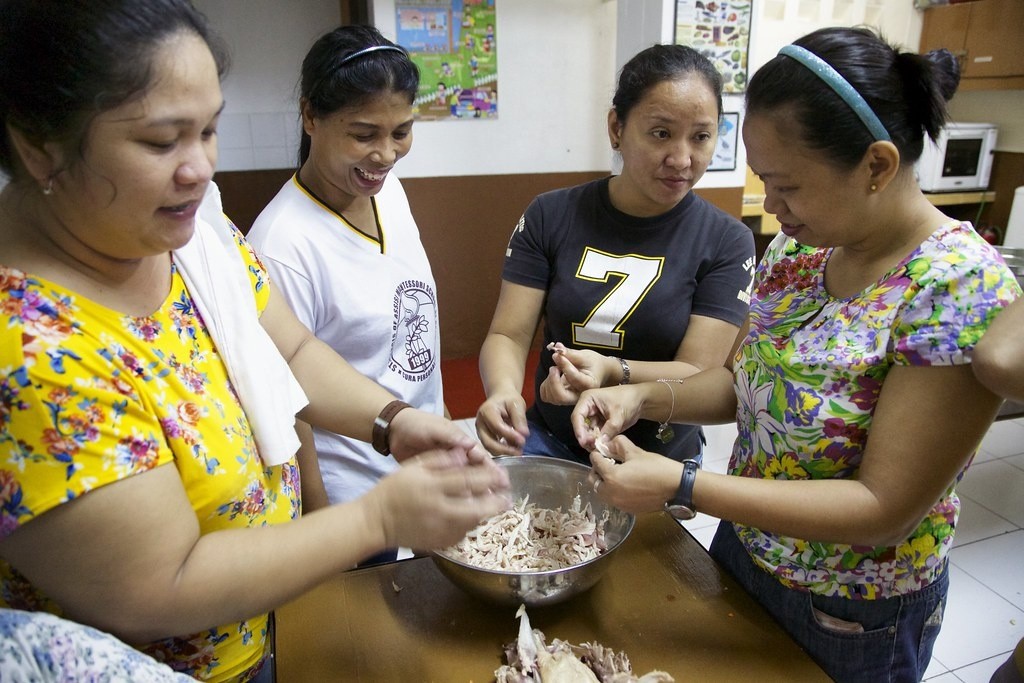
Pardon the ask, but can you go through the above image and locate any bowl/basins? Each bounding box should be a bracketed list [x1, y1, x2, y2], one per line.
[425, 454, 636, 612]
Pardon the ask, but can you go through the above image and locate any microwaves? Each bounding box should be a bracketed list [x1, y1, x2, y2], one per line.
[914, 122, 999, 193]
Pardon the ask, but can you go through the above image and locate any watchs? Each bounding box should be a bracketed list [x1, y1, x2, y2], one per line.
[665, 458, 703, 520]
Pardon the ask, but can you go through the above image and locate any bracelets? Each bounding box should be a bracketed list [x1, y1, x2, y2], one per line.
[615, 357, 630, 387]
[656, 379, 684, 444]
[373, 401, 412, 456]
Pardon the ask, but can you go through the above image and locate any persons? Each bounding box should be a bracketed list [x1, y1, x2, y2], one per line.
[1, 1, 513, 683]
[974, 296, 1024, 682]
[475, 44, 756, 468]
[240, 25, 454, 570]
[571, 27, 1023, 683]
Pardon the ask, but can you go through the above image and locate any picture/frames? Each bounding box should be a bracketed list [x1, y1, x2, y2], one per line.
[707, 112, 739, 171]
[673, 0, 753, 97]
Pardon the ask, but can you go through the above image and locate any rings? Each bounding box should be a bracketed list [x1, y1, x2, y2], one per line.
[593, 480, 604, 494]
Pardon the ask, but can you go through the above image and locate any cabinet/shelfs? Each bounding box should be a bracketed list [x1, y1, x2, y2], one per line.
[918, 0, 1024, 92]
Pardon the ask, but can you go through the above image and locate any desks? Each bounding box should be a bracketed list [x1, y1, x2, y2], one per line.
[744, 190, 998, 235]
[270, 418, 835, 683]
